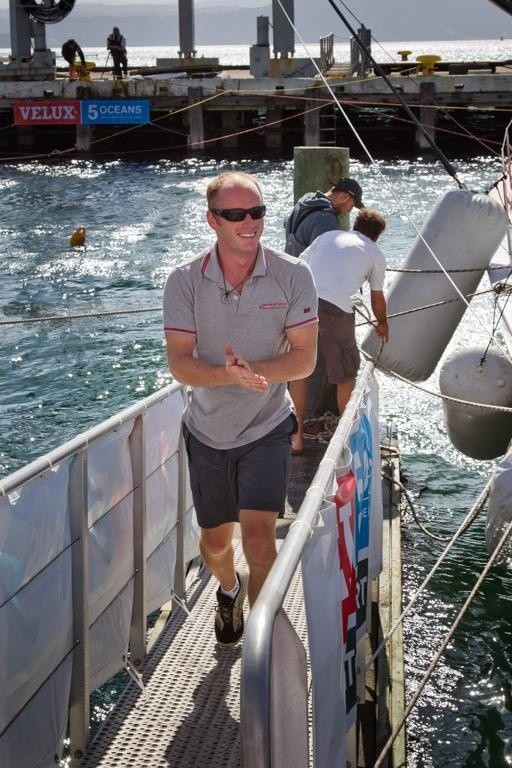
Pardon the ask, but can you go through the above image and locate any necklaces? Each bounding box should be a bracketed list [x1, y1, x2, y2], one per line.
[219, 264, 254, 304]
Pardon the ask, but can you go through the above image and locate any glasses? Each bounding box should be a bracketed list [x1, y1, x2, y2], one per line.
[209, 206, 265, 221]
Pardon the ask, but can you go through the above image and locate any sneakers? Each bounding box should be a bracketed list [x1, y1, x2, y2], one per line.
[215, 572, 248, 643]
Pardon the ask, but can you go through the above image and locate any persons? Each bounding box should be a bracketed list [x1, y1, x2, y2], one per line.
[161, 170, 320, 652]
[106, 27, 128, 80]
[282, 177, 367, 257]
[61, 39, 87, 78]
[289, 209, 389, 455]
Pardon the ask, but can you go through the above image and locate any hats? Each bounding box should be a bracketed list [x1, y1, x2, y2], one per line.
[332, 177, 365, 209]
